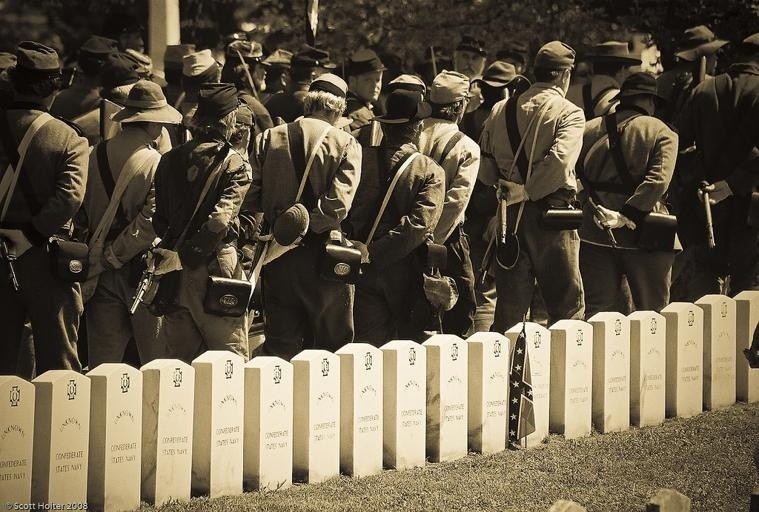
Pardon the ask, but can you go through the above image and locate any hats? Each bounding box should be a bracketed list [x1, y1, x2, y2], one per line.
[1, 23, 759, 124]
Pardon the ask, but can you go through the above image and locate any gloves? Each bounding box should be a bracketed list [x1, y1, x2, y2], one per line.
[0, 228, 33, 261]
[151, 246, 184, 277]
[351, 239, 370, 265]
[86, 252, 107, 280]
[698, 179, 734, 206]
[495, 178, 529, 207]
[257, 233, 300, 266]
[597, 204, 627, 229]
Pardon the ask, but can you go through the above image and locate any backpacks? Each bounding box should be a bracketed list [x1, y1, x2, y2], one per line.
[638, 211, 678, 254]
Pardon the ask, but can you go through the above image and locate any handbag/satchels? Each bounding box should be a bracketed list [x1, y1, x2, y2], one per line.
[420, 239, 449, 274]
[201, 275, 252, 318]
[539, 199, 585, 233]
[318, 242, 362, 285]
[46, 236, 91, 292]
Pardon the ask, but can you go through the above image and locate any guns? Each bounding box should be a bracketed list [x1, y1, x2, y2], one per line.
[585, 195, 619, 247]
[0, 237, 21, 289]
[130, 252, 161, 314]
[499, 173, 509, 246]
[130, 246, 159, 312]
